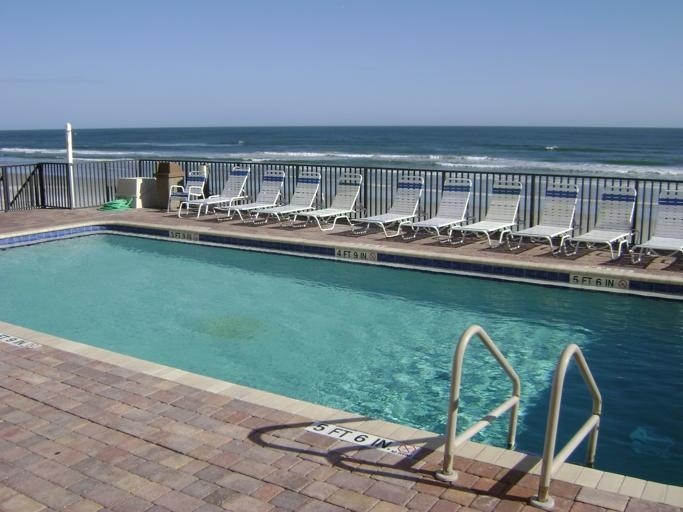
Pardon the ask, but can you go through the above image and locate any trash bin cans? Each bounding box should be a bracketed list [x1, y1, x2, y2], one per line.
[157, 162, 185, 209]
